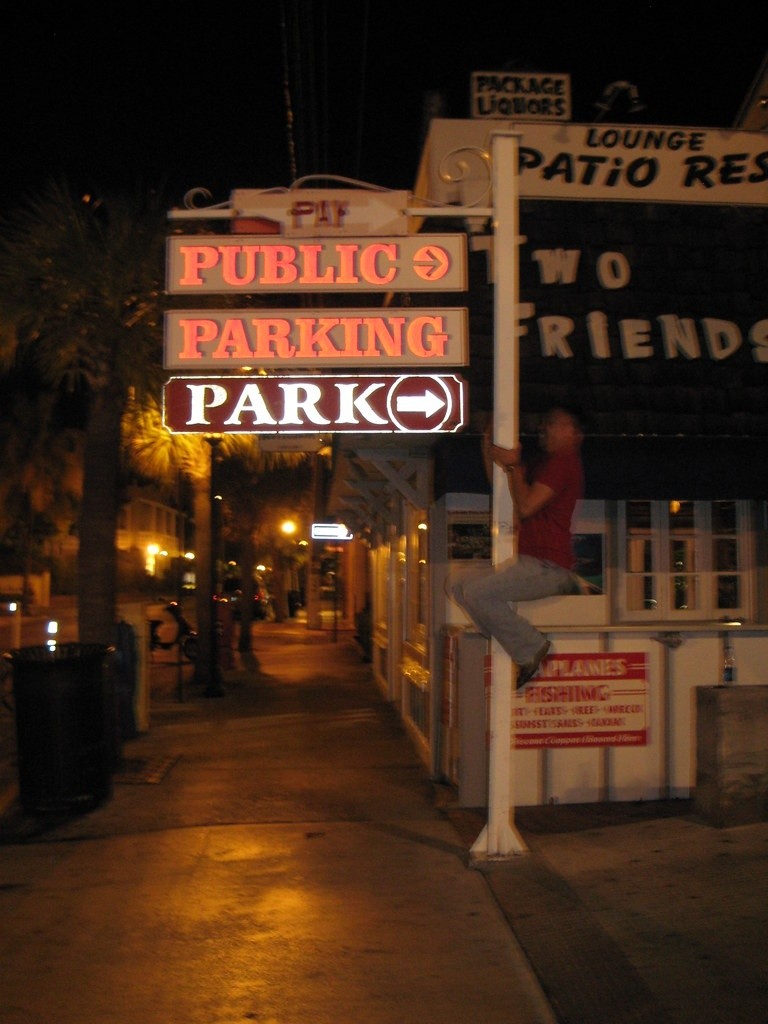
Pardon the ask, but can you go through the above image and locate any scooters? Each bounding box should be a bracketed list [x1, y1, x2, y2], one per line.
[145, 595, 201, 661]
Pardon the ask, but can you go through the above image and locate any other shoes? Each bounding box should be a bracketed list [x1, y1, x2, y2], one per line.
[515, 638, 552, 690]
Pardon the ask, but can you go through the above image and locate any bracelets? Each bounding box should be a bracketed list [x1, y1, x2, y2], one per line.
[506, 463, 521, 472]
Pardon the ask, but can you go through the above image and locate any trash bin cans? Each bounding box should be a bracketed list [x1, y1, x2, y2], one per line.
[11, 644, 115, 814]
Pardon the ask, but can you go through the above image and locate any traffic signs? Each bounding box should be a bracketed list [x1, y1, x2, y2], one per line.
[165, 234, 469, 294]
[421, 117, 768, 205]
[163, 306, 471, 366]
[165, 373, 470, 436]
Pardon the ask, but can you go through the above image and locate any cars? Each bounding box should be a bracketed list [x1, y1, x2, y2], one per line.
[211, 574, 269, 622]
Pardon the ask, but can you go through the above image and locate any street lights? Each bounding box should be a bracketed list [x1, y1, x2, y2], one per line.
[282, 518, 297, 616]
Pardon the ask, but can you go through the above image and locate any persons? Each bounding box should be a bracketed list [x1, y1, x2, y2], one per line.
[444, 408, 582, 689]
